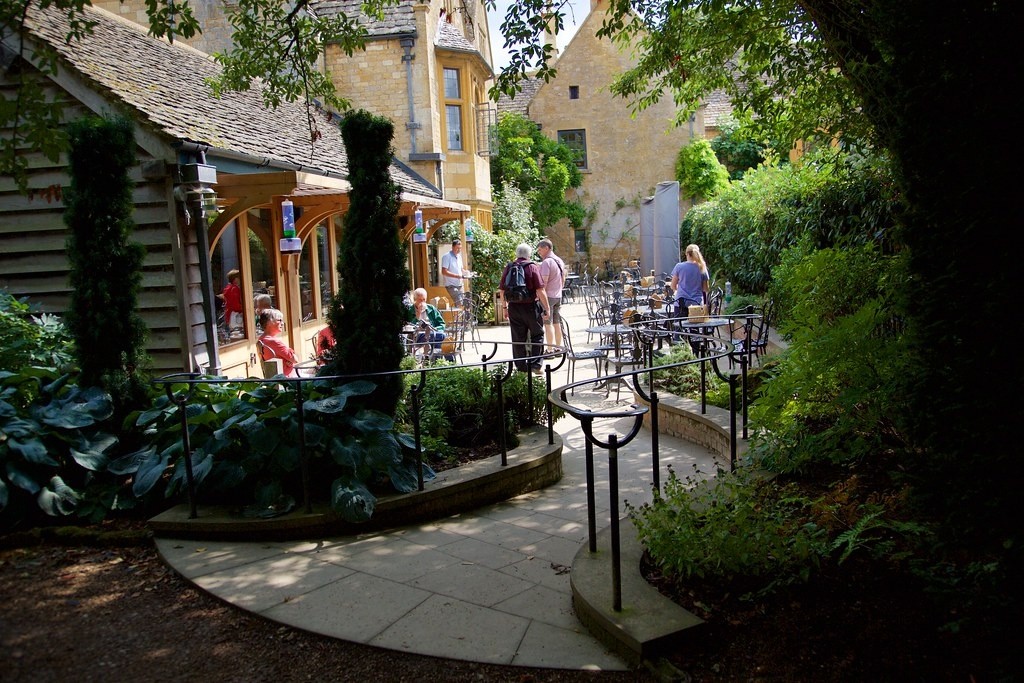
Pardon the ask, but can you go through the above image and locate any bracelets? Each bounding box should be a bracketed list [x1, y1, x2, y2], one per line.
[503, 307, 507, 309]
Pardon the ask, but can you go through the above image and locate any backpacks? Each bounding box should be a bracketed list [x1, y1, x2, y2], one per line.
[504, 262, 536, 302]
[230, 311, 245, 331]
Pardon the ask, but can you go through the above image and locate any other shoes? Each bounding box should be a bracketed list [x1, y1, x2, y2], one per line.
[531, 368, 543, 376]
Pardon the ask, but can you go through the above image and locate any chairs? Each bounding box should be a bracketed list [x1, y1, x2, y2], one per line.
[732, 298, 774, 364]
[572, 264, 600, 301]
[443, 291, 482, 353]
[584, 275, 632, 326]
[707, 305, 760, 370]
[556, 316, 606, 396]
[256, 340, 276, 380]
[706, 289, 724, 360]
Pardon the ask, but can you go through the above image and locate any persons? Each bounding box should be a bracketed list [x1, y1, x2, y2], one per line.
[257, 309, 315, 377]
[670, 243, 711, 341]
[536, 239, 566, 359]
[222, 269, 276, 335]
[498, 244, 550, 375]
[409, 288, 448, 350]
[442, 239, 468, 308]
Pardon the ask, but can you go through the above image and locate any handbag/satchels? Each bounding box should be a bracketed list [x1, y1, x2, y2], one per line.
[441, 337, 454, 354]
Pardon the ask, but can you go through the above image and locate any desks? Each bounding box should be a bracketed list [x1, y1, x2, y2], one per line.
[565, 275, 580, 302]
[585, 325, 638, 404]
[440, 306, 462, 314]
[623, 285, 675, 318]
[673, 318, 734, 366]
[217, 319, 245, 346]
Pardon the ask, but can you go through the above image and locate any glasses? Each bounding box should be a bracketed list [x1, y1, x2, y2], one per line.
[276, 319, 283, 323]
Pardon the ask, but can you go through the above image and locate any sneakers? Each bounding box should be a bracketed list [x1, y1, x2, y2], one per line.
[554, 348, 562, 357]
[543, 349, 554, 358]
